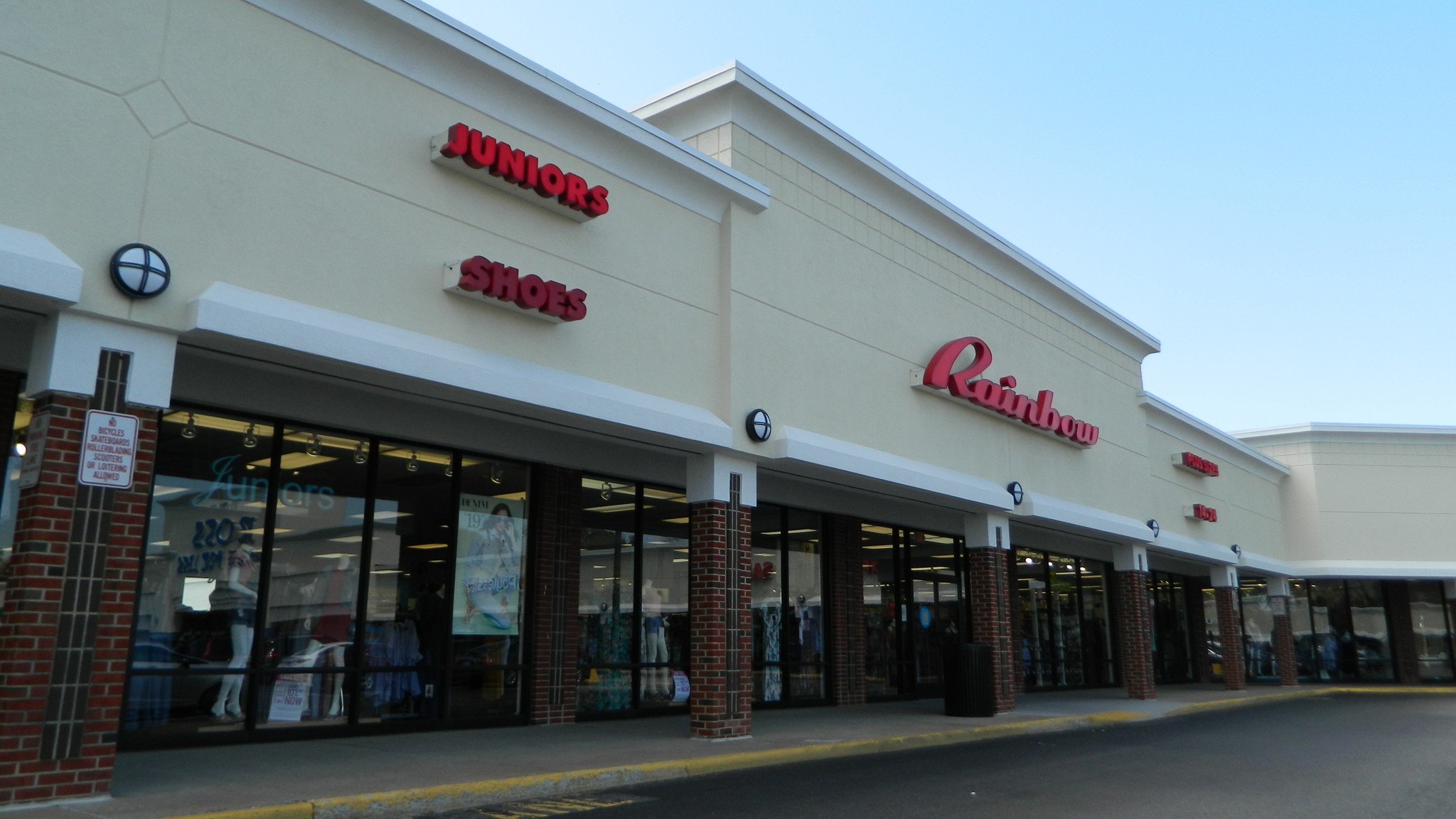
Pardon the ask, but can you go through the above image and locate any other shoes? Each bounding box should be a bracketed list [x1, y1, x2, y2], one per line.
[462, 597, 475, 625]
[509, 612, 518, 623]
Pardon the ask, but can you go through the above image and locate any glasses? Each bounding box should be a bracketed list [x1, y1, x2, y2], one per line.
[496, 517, 509, 525]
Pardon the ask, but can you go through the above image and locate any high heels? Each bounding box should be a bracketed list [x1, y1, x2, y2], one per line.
[323, 707, 342, 720]
[643, 686, 664, 700]
[660, 690, 674, 700]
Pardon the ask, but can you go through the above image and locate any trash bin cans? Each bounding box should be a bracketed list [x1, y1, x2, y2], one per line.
[941, 642, 996, 718]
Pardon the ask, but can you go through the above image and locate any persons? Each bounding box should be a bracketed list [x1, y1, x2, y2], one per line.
[462, 502, 522, 630]
[642, 579, 675, 697]
[210, 544, 362, 726]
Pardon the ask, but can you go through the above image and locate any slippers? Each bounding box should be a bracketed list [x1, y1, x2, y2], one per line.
[231, 711, 245, 723]
[209, 713, 234, 724]
